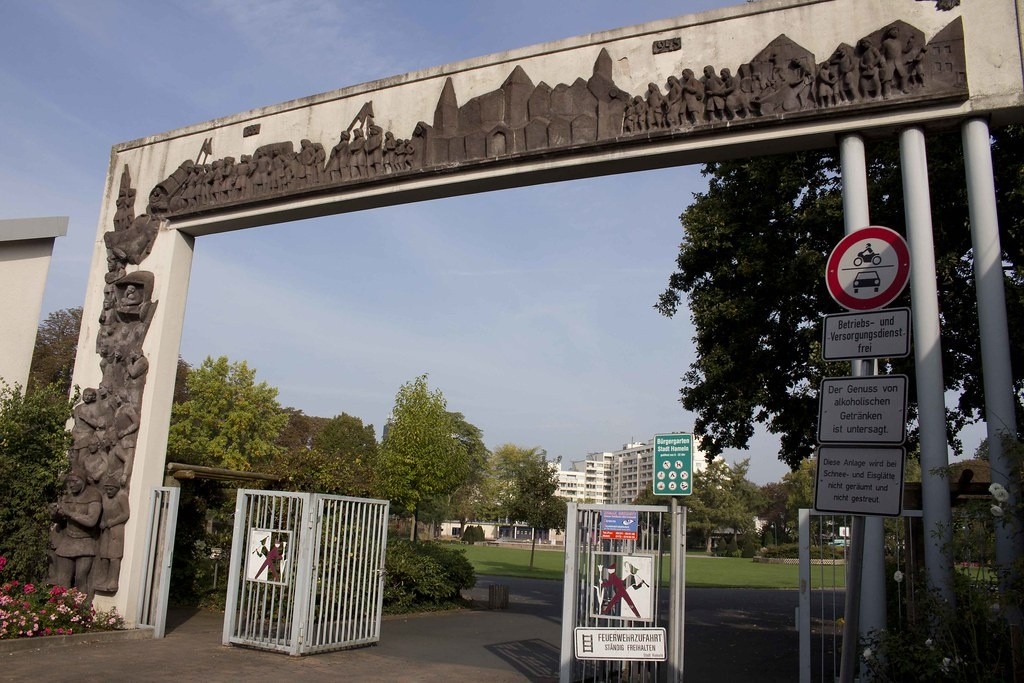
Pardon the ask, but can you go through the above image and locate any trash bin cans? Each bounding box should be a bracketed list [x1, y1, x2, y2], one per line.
[488, 583, 511, 609]
